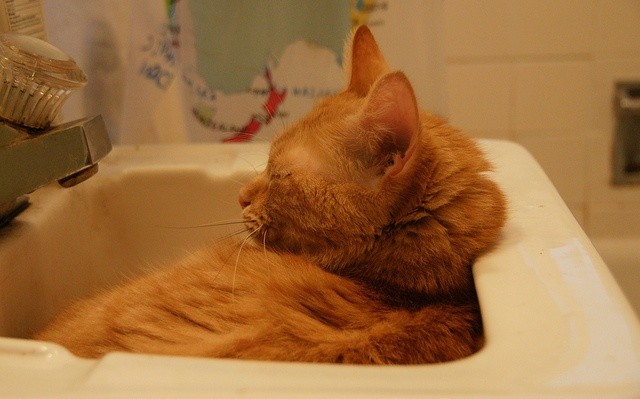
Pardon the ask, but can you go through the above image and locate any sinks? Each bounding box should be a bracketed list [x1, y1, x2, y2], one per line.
[0, 138, 640, 398]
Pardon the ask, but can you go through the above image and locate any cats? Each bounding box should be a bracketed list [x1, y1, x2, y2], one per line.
[34, 23, 507, 365]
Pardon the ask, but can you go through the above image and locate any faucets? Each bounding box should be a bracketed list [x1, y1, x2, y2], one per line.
[1, 33, 112, 226]
